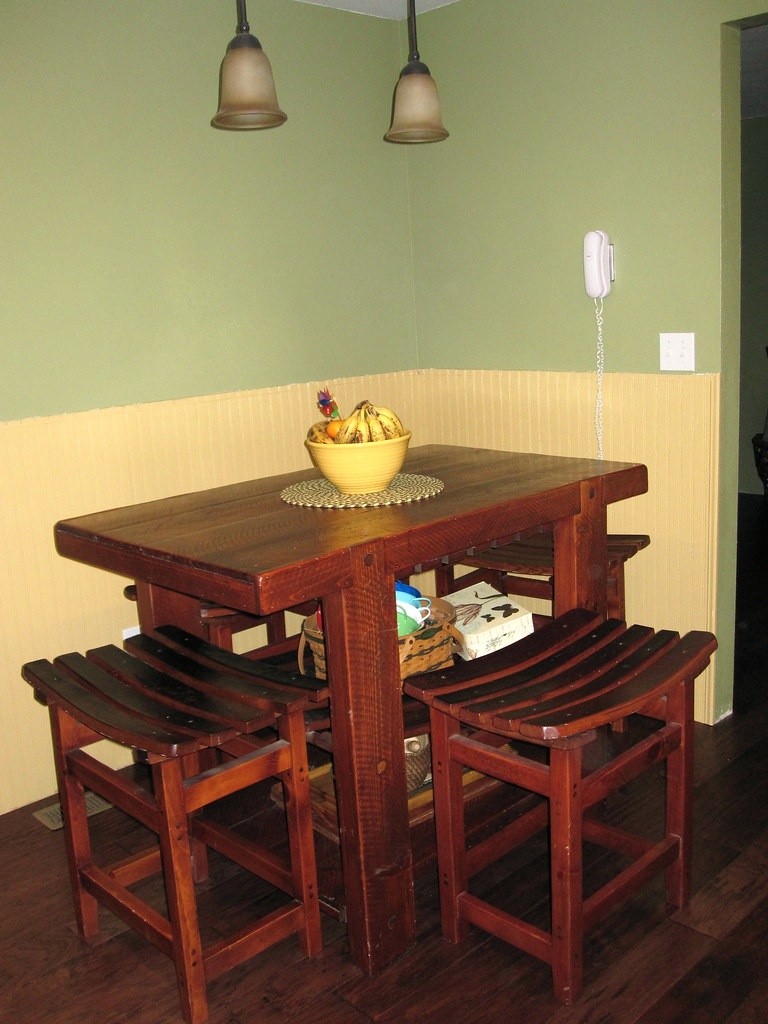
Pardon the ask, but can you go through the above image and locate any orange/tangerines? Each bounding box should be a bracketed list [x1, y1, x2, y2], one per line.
[326, 419, 346, 438]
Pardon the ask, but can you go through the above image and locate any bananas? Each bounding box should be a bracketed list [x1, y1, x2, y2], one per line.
[307, 398, 404, 444]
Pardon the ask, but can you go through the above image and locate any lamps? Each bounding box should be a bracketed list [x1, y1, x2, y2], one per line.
[383, 0, 450, 146]
[210, 0, 287, 132]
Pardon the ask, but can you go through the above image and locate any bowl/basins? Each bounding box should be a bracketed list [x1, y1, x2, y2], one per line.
[395, 580, 431, 636]
[307, 432, 413, 495]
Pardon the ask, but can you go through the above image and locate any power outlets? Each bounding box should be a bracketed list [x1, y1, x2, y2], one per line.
[659, 333, 694, 372]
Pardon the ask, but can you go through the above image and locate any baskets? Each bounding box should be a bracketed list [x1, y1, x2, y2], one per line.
[404, 734, 431, 793]
[297, 594, 468, 696]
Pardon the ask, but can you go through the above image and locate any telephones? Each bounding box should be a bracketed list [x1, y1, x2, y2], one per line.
[584, 230, 611, 299]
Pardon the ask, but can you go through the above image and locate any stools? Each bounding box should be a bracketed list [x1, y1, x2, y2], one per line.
[434, 533, 651, 734]
[21, 624, 331, 1024]
[402, 610, 718, 1007]
[123, 576, 415, 765]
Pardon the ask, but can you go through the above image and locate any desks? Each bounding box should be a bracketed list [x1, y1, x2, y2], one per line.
[55, 443, 649, 976]
